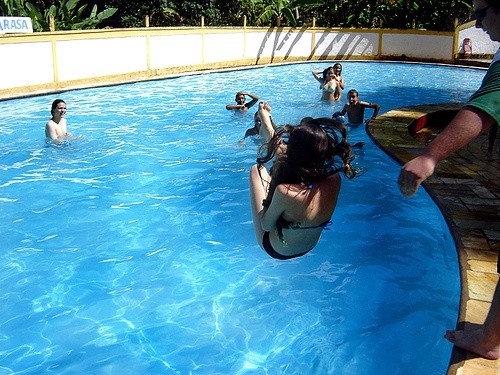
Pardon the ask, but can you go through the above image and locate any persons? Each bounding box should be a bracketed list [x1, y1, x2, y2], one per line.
[225, 89, 258, 115]
[45, 98, 83, 145]
[312, 63, 346, 101]
[397, 0, 500, 361]
[332, 89, 380, 130]
[242, 112, 281, 141]
[249, 99, 367, 261]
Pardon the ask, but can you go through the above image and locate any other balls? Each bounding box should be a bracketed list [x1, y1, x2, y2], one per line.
[337, 116, 344, 122]
